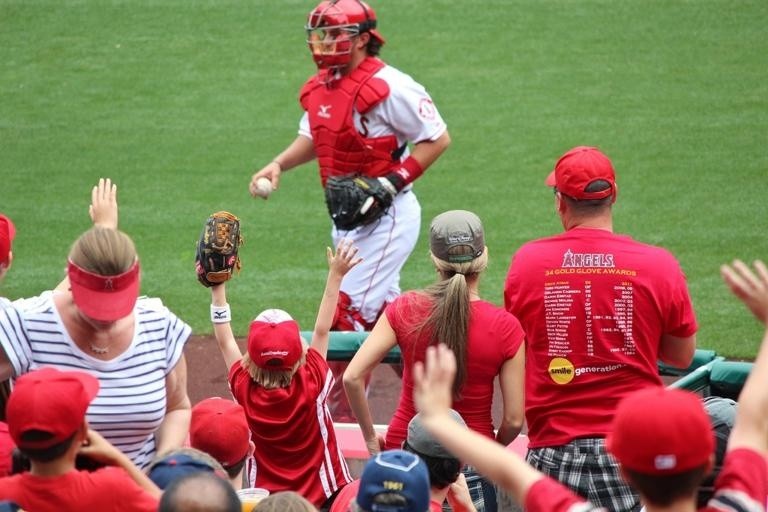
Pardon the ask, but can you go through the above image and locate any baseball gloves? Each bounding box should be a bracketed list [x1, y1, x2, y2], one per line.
[195, 211, 243, 287]
[326, 172, 395, 230]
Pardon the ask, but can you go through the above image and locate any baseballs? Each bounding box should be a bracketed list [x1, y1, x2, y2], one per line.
[254, 176, 272, 198]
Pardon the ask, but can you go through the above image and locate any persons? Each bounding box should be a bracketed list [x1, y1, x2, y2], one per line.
[247, 1, 454, 423]
[3, 145, 768, 512]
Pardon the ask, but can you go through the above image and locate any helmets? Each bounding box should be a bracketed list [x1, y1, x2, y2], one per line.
[304, 0, 385, 87]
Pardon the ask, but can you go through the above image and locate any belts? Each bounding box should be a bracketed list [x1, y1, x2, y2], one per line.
[549, 445, 607, 455]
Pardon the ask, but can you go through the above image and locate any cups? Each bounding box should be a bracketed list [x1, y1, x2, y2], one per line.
[236, 487, 270, 512]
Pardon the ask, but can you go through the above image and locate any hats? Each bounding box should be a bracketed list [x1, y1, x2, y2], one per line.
[407, 407, 468, 459]
[0, 213, 15, 265]
[545, 145, 616, 199]
[604, 387, 717, 475]
[67, 253, 142, 322]
[430, 209, 485, 263]
[247, 309, 303, 371]
[189, 396, 253, 469]
[147, 453, 215, 490]
[4, 366, 100, 449]
[356, 449, 432, 512]
